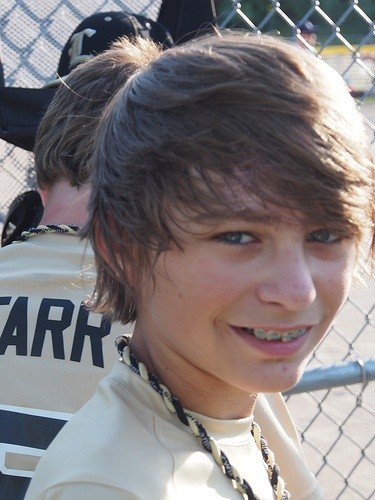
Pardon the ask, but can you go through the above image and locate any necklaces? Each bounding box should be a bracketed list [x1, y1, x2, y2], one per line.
[21, 223, 88, 241]
[114, 334, 291, 500]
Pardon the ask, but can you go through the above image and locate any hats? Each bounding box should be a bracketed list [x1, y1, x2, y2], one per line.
[44, 12, 177, 90]
[299, 20, 318, 32]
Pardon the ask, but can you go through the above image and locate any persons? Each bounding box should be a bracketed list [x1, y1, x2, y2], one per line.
[0, 34, 165, 500]
[25, 30, 375, 500]
